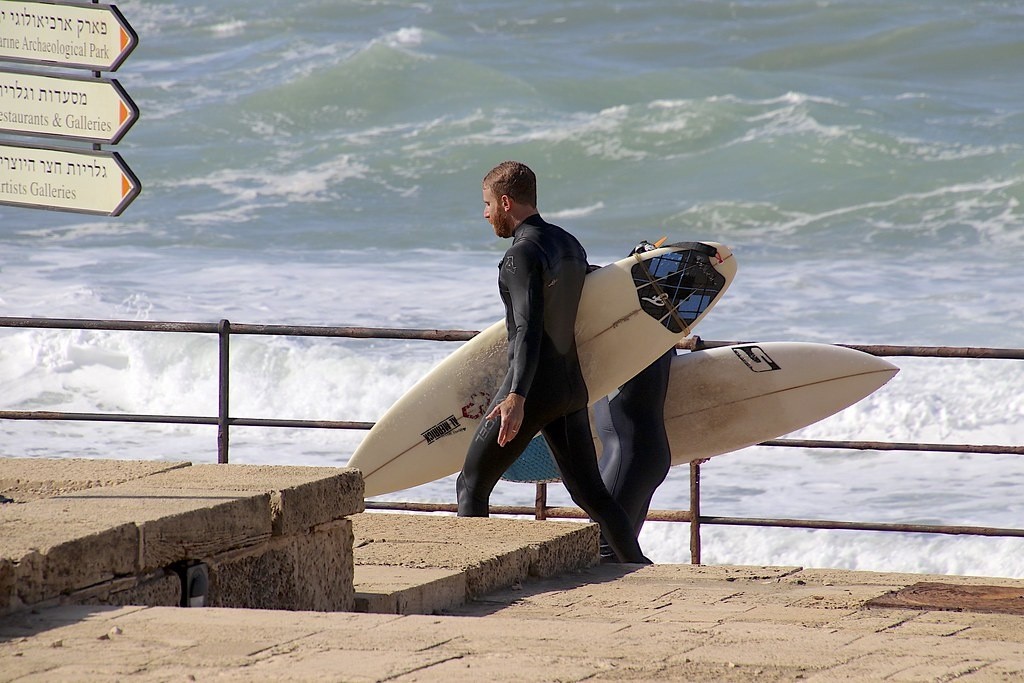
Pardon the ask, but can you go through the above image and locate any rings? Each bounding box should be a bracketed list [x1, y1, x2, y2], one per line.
[513, 430, 516, 432]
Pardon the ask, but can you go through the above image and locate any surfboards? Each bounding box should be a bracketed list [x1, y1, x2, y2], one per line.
[345, 240, 737, 498]
[499, 340, 901, 485]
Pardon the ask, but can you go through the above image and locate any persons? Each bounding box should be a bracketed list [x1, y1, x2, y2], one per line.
[456, 161, 653, 564]
[589, 240, 677, 564]
